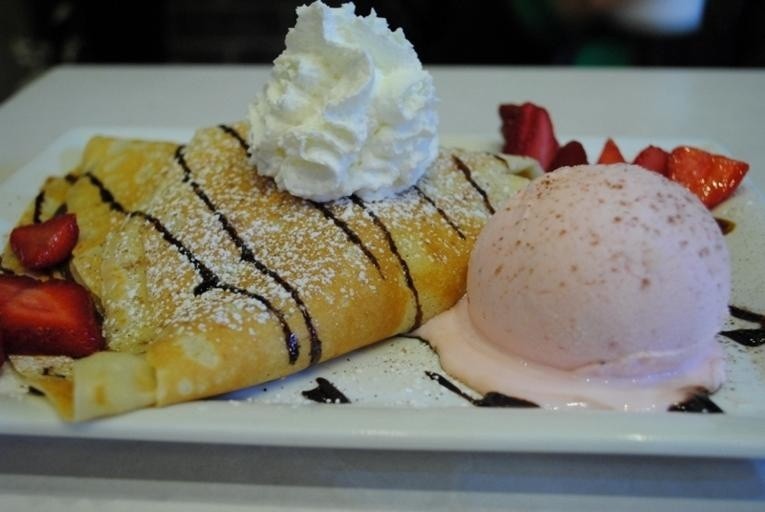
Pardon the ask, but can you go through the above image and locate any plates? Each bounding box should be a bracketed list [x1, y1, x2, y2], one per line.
[0, 63, 765, 459]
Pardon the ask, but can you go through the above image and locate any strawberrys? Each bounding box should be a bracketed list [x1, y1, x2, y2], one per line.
[0, 213, 110, 359]
[497, 102, 748, 211]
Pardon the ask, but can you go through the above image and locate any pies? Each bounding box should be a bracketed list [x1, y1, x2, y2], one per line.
[0, 134, 544, 424]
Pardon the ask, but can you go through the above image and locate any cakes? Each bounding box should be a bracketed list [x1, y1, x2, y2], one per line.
[247, 1, 442, 203]
[410, 161, 735, 412]
